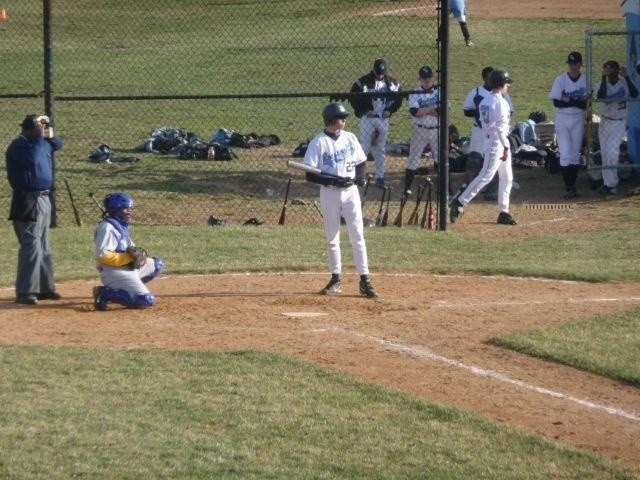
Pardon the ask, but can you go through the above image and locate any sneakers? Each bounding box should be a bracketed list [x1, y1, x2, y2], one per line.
[16, 295, 38, 304]
[322, 274, 342, 295]
[93, 286, 106, 310]
[40, 292, 61, 299]
[497, 212, 517, 225]
[359, 275, 378, 298]
[450, 199, 464, 223]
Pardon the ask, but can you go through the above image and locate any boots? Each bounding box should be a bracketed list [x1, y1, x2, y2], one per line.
[405, 169, 417, 195]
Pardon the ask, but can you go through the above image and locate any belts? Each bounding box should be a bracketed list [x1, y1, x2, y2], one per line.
[418, 125, 440, 129]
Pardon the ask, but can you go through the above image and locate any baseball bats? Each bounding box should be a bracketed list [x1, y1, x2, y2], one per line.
[361, 174, 435, 229]
[288, 161, 366, 187]
[64, 181, 82, 228]
[279, 179, 290, 224]
[89, 192, 109, 218]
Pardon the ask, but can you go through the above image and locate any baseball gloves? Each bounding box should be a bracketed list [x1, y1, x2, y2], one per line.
[243, 217, 264, 225]
[208, 214, 227, 225]
[126, 247, 148, 269]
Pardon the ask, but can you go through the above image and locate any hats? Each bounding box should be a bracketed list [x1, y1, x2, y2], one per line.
[419, 66, 432, 77]
[567, 52, 582, 63]
[374, 59, 387, 75]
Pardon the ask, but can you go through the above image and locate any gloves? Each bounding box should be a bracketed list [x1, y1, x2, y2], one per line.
[336, 177, 352, 188]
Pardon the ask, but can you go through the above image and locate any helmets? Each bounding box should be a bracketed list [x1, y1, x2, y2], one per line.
[322, 102, 350, 126]
[22, 115, 54, 138]
[103, 193, 133, 229]
[487, 70, 513, 89]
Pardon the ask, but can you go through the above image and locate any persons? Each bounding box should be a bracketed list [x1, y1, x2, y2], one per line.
[403, 65, 440, 194]
[593, 59, 639, 199]
[549, 51, 593, 200]
[461, 66, 522, 190]
[451, 70, 519, 226]
[435, 0, 477, 47]
[620, 0, 640, 72]
[348, 58, 403, 190]
[302, 102, 379, 299]
[625, 57, 640, 185]
[4, 113, 63, 306]
[91, 192, 165, 311]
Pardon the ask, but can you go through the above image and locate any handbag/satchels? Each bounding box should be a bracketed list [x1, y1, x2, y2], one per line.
[8, 190, 38, 221]
